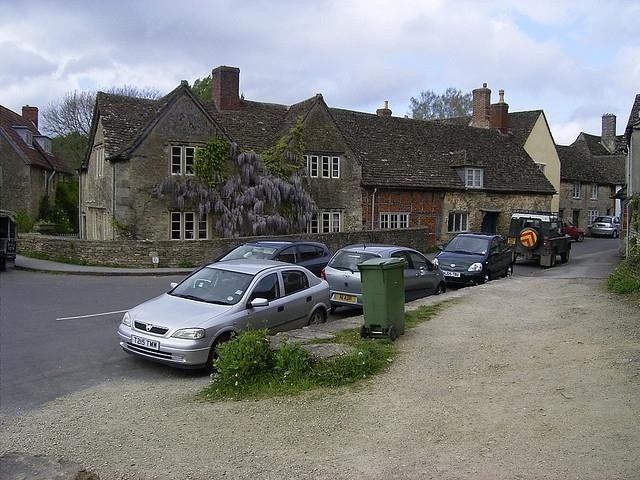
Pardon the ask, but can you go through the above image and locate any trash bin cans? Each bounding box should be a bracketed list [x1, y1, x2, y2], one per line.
[356, 258, 408, 341]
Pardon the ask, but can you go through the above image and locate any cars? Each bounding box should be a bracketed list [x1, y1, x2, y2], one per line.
[212, 239, 332, 279]
[428, 232, 515, 291]
[118, 260, 332, 376]
[563, 221, 585, 242]
[321, 242, 446, 322]
[590, 215, 621, 238]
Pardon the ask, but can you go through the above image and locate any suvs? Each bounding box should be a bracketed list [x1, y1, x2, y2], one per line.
[506, 208, 571, 267]
